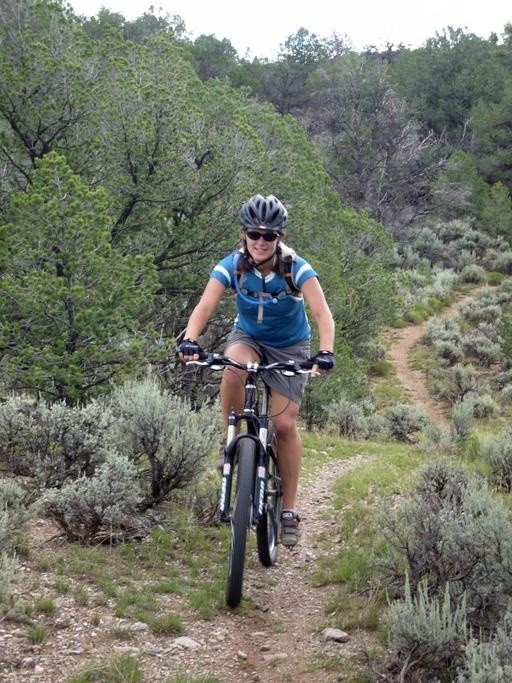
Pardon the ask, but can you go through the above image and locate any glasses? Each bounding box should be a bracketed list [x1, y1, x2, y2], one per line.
[245, 231, 280, 242]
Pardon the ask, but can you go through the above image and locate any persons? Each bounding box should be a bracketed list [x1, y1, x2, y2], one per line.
[178, 193, 336, 546]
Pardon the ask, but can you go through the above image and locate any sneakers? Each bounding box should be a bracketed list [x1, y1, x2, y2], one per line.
[280, 511, 300, 546]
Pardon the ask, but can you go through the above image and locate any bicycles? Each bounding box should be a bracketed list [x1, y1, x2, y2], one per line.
[176, 346, 335, 609]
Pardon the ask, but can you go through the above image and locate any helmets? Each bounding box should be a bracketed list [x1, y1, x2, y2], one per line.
[238, 193, 288, 231]
